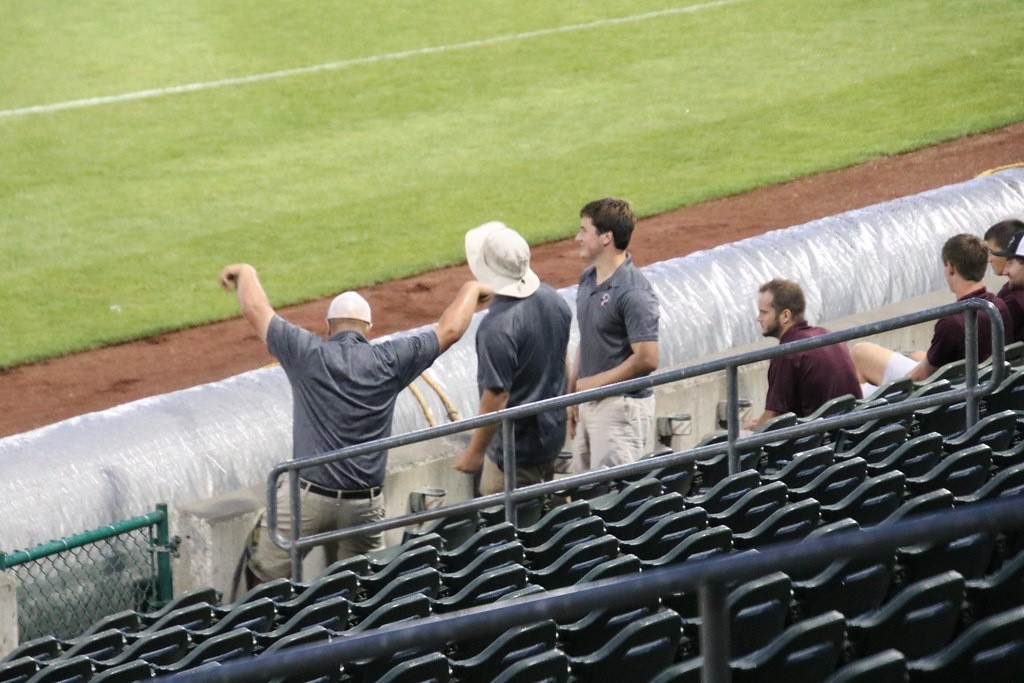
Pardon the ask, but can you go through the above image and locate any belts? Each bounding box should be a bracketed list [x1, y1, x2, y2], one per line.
[299, 481, 381, 499]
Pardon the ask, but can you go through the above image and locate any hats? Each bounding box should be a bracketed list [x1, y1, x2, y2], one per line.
[991, 230, 1024, 257]
[465, 222, 540, 298]
[327, 291, 372, 324]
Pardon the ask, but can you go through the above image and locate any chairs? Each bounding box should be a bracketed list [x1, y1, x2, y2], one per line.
[0, 340, 1024, 683]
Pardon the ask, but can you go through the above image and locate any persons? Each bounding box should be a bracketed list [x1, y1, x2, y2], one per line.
[984, 219, 1024, 344]
[740, 279, 863, 432]
[567, 198, 660, 478]
[849, 234, 1014, 397]
[451, 221, 571, 496]
[219, 263, 492, 595]
